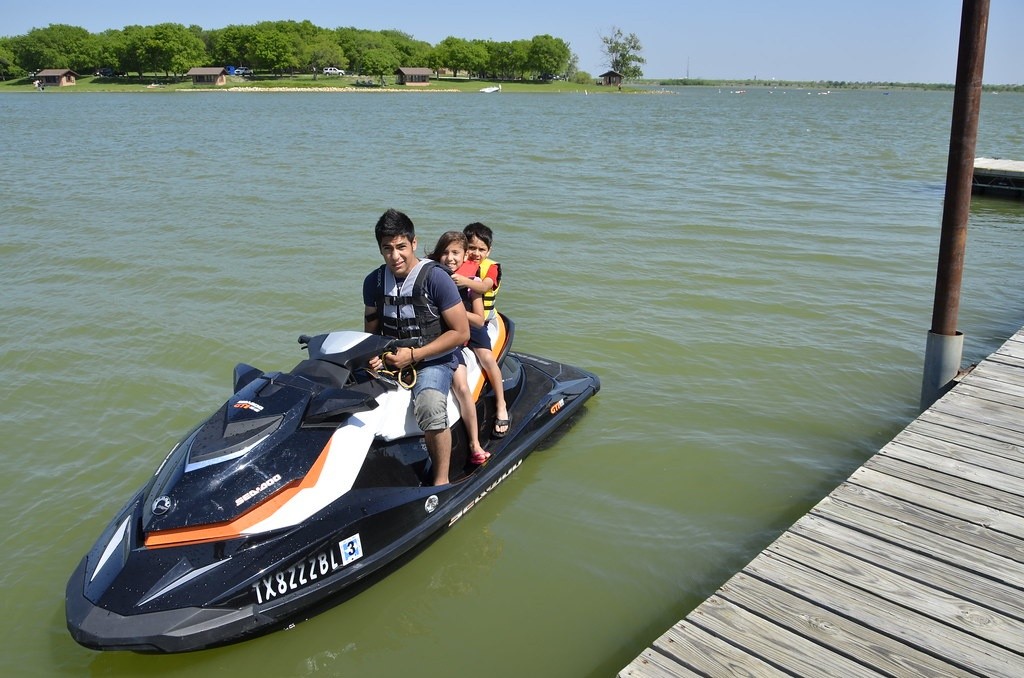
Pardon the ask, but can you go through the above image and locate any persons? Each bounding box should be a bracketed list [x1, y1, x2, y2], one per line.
[363, 209, 471, 486]
[423, 230, 492, 464]
[462, 222, 512, 438]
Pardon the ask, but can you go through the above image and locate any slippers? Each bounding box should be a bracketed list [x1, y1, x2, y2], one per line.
[471, 452, 491, 464]
[493, 411, 513, 438]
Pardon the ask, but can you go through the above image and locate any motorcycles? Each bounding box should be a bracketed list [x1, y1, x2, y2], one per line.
[64, 331, 601, 655]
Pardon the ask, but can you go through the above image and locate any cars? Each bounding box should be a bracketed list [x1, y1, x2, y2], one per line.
[538, 73, 560, 80]
[94, 67, 125, 77]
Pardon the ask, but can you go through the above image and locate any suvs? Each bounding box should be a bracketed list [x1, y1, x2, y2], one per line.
[322, 67, 345, 76]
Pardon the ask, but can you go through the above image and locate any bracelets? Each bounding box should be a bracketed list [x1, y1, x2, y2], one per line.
[411, 348, 417, 364]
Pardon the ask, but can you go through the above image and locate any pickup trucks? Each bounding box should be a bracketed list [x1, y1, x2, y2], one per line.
[234, 67, 254, 77]
